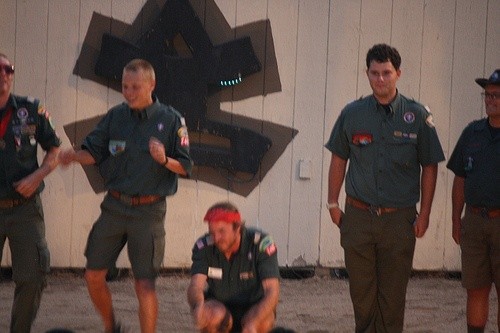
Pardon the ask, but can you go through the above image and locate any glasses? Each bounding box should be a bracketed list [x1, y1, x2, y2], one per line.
[5, 65, 15, 74]
[480, 91, 499, 99]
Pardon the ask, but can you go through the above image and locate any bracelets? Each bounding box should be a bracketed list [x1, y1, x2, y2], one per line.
[161, 157, 169, 167]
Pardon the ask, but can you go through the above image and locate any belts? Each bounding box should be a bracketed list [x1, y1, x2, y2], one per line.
[471, 207, 499, 219]
[0, 199, 21, 209]
[346, 196, 400, 217]
[108, 189, 162, 208]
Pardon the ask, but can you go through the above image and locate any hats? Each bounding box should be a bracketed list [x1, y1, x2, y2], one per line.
[474, 69, 500, 87]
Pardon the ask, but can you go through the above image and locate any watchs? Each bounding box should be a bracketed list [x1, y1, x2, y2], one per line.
[325, 203, 339, 209]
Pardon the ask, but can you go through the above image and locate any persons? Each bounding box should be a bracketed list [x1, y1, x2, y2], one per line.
[54, 58, 194, 333]
[446, 68, 500, 333]
[0, 54, 61, 333]
[187, 202, 282, 333]
[324, 43, 445, 333]
